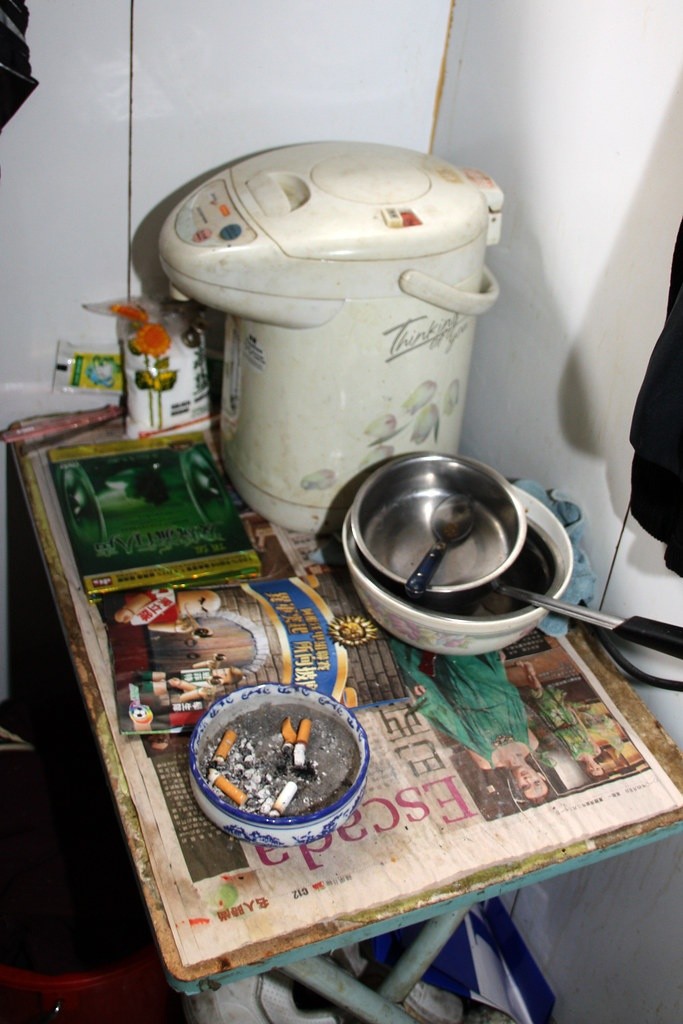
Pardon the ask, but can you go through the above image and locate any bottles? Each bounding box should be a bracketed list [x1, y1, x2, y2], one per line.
[156, 281, 202, 338]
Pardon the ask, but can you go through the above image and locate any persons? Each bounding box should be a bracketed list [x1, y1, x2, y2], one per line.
[388, 630, 549, 806]
[515, 658, 609, 782]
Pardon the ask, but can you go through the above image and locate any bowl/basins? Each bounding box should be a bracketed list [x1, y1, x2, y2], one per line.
[342, 486, 574, 655]
[349, 516, 563, 621]
[189, 683, 370, 846]
[352, 452, 527, 592]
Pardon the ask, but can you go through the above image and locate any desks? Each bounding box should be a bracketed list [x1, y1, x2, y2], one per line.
[0, 406, 683, 1024]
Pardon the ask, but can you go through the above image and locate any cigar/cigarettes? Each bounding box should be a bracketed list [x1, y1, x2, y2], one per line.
[208, 716, 313, 817]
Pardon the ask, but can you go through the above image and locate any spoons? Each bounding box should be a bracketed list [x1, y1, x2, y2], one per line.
[405, 494, 477, 598]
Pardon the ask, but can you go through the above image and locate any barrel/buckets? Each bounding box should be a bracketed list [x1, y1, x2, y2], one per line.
[0, 941, 170, 1024]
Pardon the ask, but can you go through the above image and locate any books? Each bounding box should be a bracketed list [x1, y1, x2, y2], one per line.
[45, 431, 410, 738]
